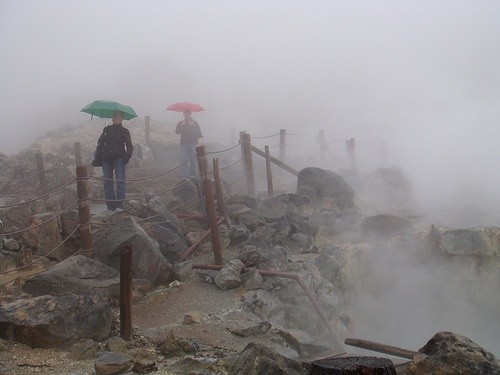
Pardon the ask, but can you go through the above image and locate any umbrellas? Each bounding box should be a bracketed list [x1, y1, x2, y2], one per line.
[80, 100, 138, 121]
[166, 101, 203, 112]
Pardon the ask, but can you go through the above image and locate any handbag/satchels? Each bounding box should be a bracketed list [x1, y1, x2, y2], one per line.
[92, 144, 104, 167]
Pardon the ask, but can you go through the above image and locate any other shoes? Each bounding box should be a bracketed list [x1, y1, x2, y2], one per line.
[103, 209, 113, 213]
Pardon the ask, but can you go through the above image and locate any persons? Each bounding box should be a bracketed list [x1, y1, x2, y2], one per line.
[98, 109, 133, 211]
[176, 110, 203, 178]
